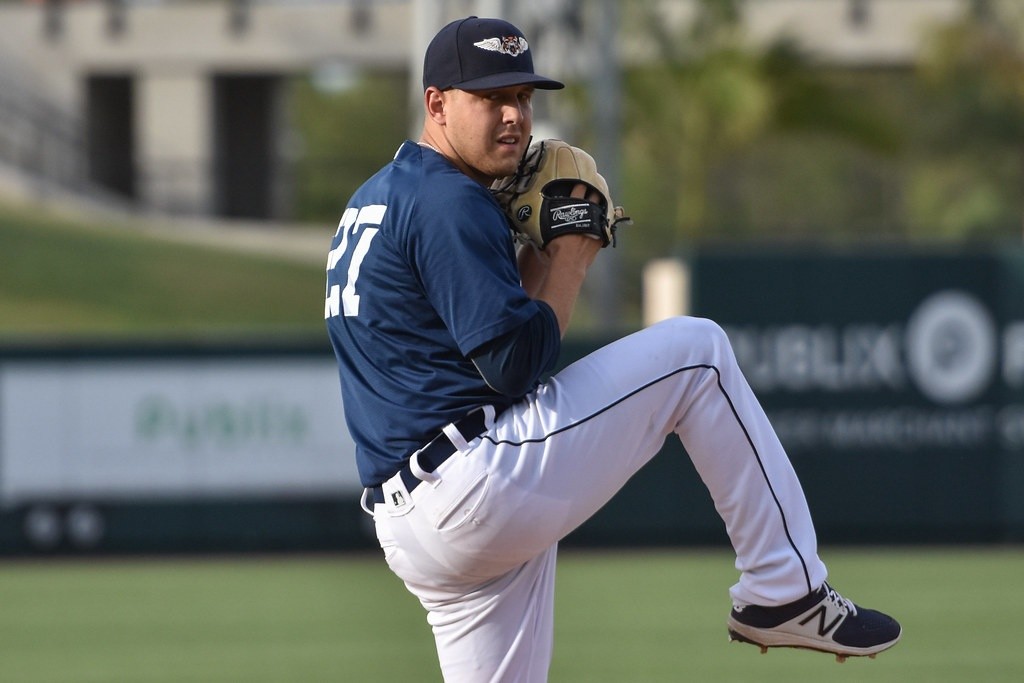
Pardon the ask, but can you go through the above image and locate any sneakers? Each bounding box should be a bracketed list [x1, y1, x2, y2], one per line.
[727, 579, 902, 663]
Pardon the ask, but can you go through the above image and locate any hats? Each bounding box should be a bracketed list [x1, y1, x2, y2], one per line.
[423, 16, 566, 91]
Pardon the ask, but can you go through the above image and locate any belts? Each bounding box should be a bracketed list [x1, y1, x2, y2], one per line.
[365, 405, 503, 512]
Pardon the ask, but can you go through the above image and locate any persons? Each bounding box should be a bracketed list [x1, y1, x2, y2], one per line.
[324, 15, 903, 683]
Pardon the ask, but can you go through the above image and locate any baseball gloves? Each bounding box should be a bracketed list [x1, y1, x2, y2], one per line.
[487, 137, 617, 251]
